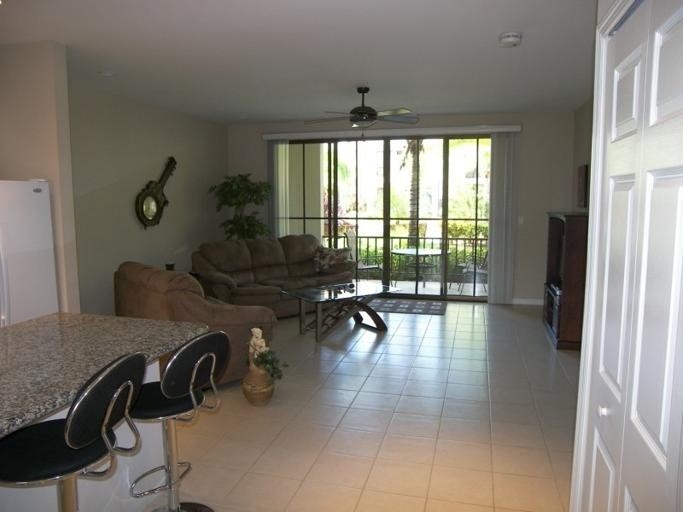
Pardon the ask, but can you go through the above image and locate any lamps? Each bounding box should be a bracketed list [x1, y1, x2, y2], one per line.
[501, 32, 525, 49]
[351, 119, 378, 141]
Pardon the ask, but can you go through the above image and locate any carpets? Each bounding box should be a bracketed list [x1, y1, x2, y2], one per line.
[361, 297, 448, 316]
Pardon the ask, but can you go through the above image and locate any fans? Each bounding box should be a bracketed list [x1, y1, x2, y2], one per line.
[312, 87, 419, 131]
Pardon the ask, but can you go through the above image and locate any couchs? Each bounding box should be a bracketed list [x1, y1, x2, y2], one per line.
[190, 234, 355, 324]
[113, 260, 290, 387]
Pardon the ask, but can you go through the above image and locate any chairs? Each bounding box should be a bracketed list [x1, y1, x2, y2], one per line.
[344, 230, 394, 289]
[2, 356, 159, 511]
[456, 246, 488, 296]
[455, 261, 470, 289]
[128, 329, 230, 511]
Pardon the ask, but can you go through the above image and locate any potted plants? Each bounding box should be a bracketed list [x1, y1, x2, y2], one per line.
[242, 340, 282, 409]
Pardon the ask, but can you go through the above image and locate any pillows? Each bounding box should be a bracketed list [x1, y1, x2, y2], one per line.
[314, 244, 351, 273]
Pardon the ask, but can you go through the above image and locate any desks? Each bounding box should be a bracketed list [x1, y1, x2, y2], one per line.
[390, 246, 451, 288]
[1, 308, 209, 447]
